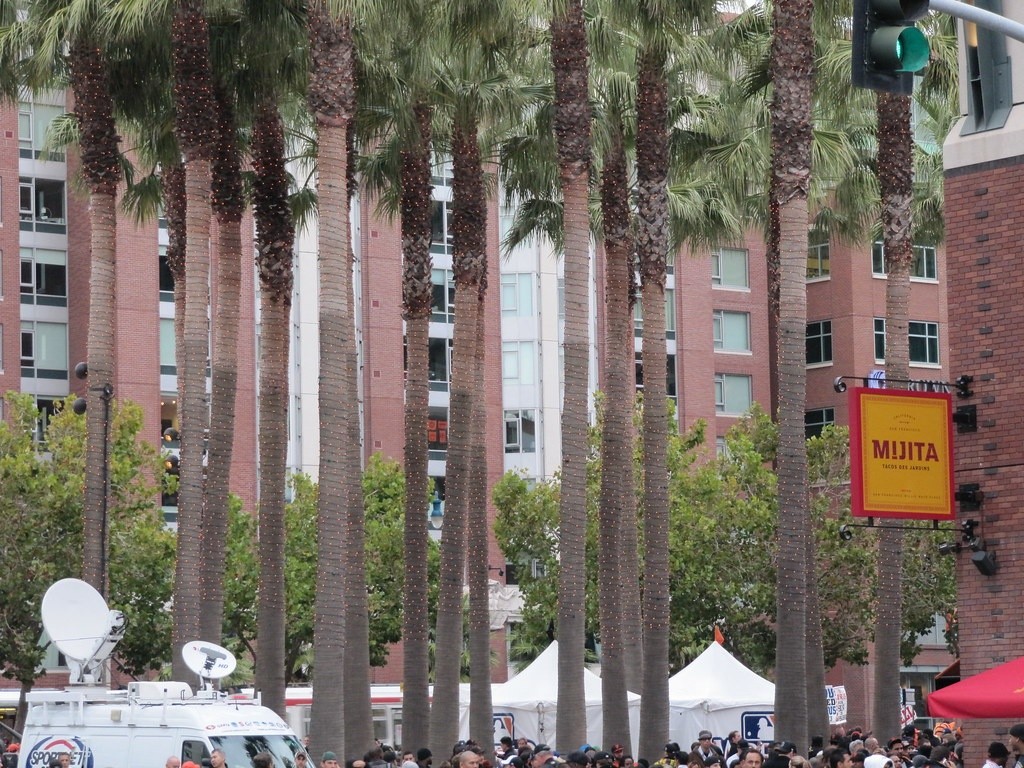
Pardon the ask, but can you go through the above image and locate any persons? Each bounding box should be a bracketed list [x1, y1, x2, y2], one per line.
[0, 722, 1024, 768]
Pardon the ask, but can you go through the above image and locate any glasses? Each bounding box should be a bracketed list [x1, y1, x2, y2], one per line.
[700, 738, 711, 740]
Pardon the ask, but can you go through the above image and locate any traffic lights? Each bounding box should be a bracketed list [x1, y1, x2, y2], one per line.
[849, 0, 937, 97]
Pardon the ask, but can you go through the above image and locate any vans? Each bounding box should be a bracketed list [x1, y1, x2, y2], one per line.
[15, 576, 319, 768]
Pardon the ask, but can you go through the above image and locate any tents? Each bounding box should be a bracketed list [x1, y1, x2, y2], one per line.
[460, 640, 776, 759]
[928, 657, 1024, 719]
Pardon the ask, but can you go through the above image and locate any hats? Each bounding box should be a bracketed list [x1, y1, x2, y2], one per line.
[913, 755, 930, 768]
[535, 743, 551, 754]
[697, 730, 712, 739]
[888, 738, 902, 748]
[705, 757, 720, 766]
[323, 751, 337, 761]
[775, 742, 797, 752]
[612, 744, 624, 752]
[562, 752, 588, 766]
[294, 751, 307, 758]
[591, 752, 609, 768]
[417, 748, 432, 760]
[1010, 724, 1024, 742]
[988, 742, 1011, 758]
